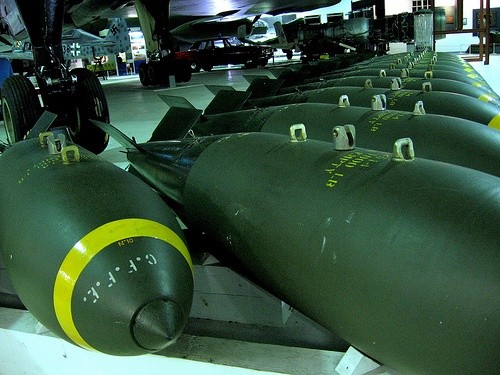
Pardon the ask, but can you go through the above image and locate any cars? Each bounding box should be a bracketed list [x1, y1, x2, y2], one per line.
[175, 36, 276, 69]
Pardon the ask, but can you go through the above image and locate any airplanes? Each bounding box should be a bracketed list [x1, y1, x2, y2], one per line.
[1, 0, 340, 156]
